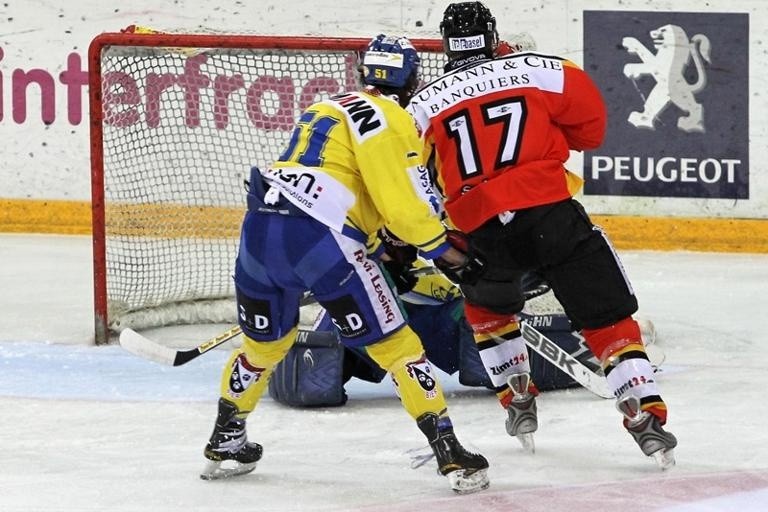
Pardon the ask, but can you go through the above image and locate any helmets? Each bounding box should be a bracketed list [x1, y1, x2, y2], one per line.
[362, 33, 420, 89]
[439, 1, 498, 60]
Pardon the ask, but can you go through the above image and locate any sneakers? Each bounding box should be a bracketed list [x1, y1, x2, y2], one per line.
[204, 397, 263, 463]
[503, 372, 538, 437]
[615, 394, 678, 457]
[416, 412, 490, 476]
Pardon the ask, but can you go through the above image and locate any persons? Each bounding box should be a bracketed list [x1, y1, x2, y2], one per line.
[403, 0, 678, 458]
[201, 31, 493, 476]
[265, 136, 668, 409]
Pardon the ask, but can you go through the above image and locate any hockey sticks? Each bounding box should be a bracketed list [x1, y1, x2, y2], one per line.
[118, 291, 314, 366]
[415, 256, 665, 400]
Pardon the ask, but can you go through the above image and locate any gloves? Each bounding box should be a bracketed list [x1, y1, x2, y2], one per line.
[384, 251, 418, 296]
